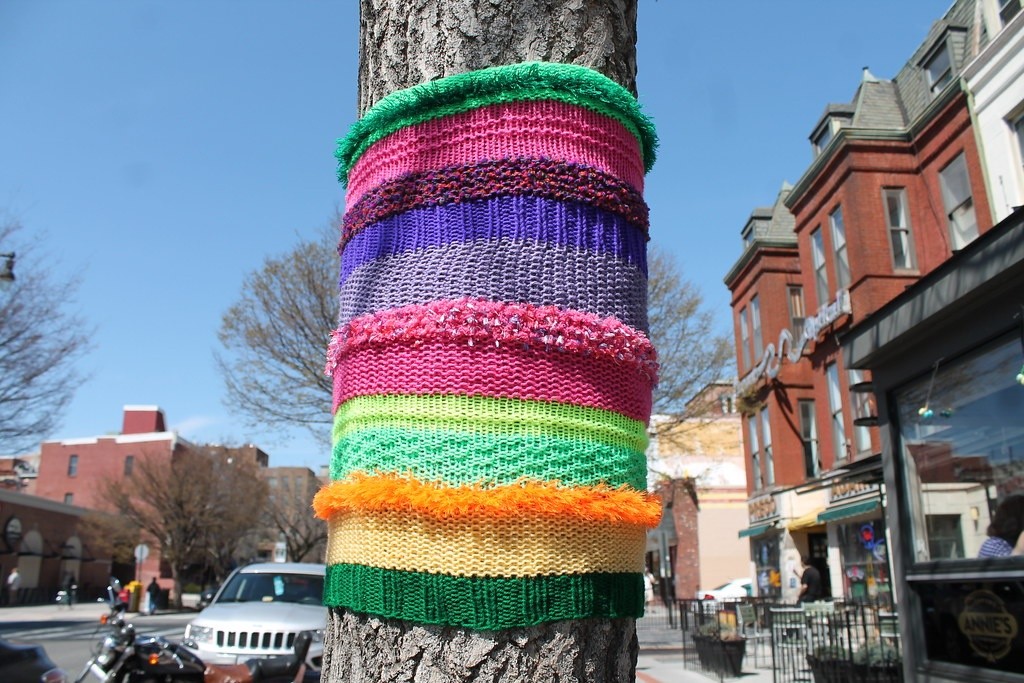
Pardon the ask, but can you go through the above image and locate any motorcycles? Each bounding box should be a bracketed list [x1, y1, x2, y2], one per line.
[69, 575, 313, 683]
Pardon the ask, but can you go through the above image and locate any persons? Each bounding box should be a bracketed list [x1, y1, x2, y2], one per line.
[977, 495, 1024, 558]
[146, 577, 161, 615]
[7, 567, 20, 606]
[643, 567, 656, 613]
[794, 555, 820, 607]
[62, 571, 76, 606]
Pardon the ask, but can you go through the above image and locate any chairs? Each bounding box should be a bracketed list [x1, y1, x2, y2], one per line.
[800, 601, 834, 656]
[769, 607, 806, 671]
[735, 603, 772, 668]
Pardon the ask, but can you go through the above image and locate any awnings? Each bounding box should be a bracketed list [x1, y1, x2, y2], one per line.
[738, 523, 770, 538]
[816, 494, 881, 524]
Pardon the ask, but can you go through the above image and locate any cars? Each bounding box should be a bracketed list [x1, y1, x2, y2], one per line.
[0, 634, 67, 683]
[179, 561, 331, 663]
[691, 580, 753, 615]
